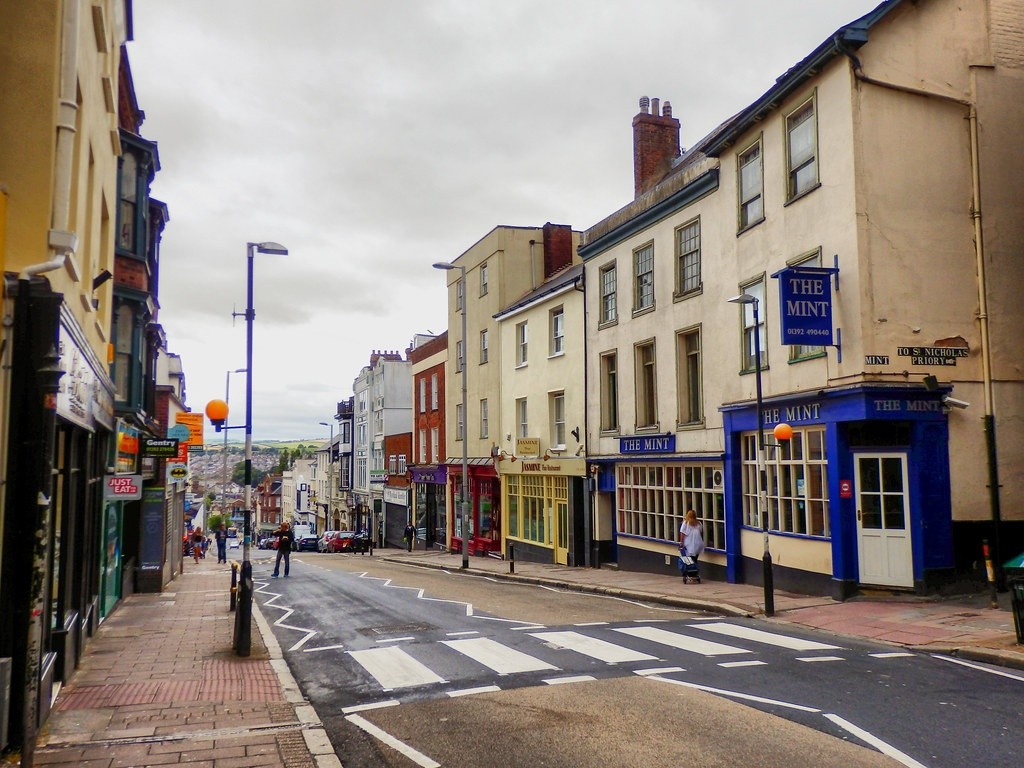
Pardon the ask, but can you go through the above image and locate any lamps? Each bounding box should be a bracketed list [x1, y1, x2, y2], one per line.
[924, 372, 940, 391]
[544, 448, 560, 461]
[411, 474, 433, 481]
[498, 450, 517, 462]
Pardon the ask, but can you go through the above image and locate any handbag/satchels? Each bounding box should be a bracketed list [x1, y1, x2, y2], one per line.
[273, 537, 280, 549]
[404, 538, 407, 544]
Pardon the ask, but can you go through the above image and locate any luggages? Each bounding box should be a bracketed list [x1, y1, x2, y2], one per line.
[678, 545, 701, 584]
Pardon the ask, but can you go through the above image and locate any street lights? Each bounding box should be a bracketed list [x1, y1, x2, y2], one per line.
[234, 242, 290, 654]
[319, 422, 332, 531]
[727, 294, 775, 617]
[221, 367, 247, 523]
[430, 261, 469, 569]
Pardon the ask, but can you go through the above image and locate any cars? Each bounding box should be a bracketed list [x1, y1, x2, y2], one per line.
[187, 532, 212, 557]
[416, 509, 492, 541]
[225, 524, 369, 554]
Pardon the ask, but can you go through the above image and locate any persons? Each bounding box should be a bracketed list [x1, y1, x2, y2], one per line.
[191, 523, 227, 564]
[270, 522, 294, 578]
[680, 510, 705, 583]
[404, 521, 417, 552]
